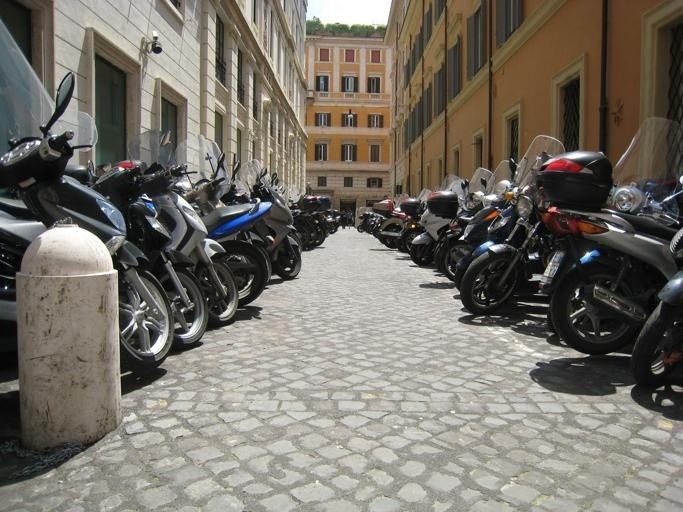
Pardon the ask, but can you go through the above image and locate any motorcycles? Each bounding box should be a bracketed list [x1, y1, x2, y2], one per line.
[630, 228, 683, 392]
[358, 115, 683, 334]
[0, 19, 175, 373]
[65, 127, 344, 351]
[531, 150, 683, 356]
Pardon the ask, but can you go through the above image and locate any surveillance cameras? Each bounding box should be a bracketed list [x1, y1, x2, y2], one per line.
[151, 31, 162, 53]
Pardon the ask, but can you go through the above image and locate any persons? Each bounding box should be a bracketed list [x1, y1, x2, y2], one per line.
[340, 209, 346, 230]
[343, 209, 354, 230]
